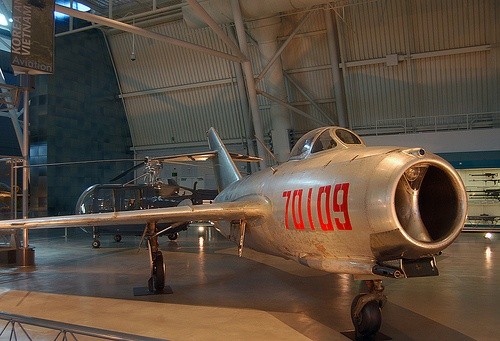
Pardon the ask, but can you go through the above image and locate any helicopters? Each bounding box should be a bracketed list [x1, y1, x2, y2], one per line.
[13, 156, 218, 248]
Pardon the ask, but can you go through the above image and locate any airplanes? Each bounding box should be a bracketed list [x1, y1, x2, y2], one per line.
[0, 127, 468, 341]
